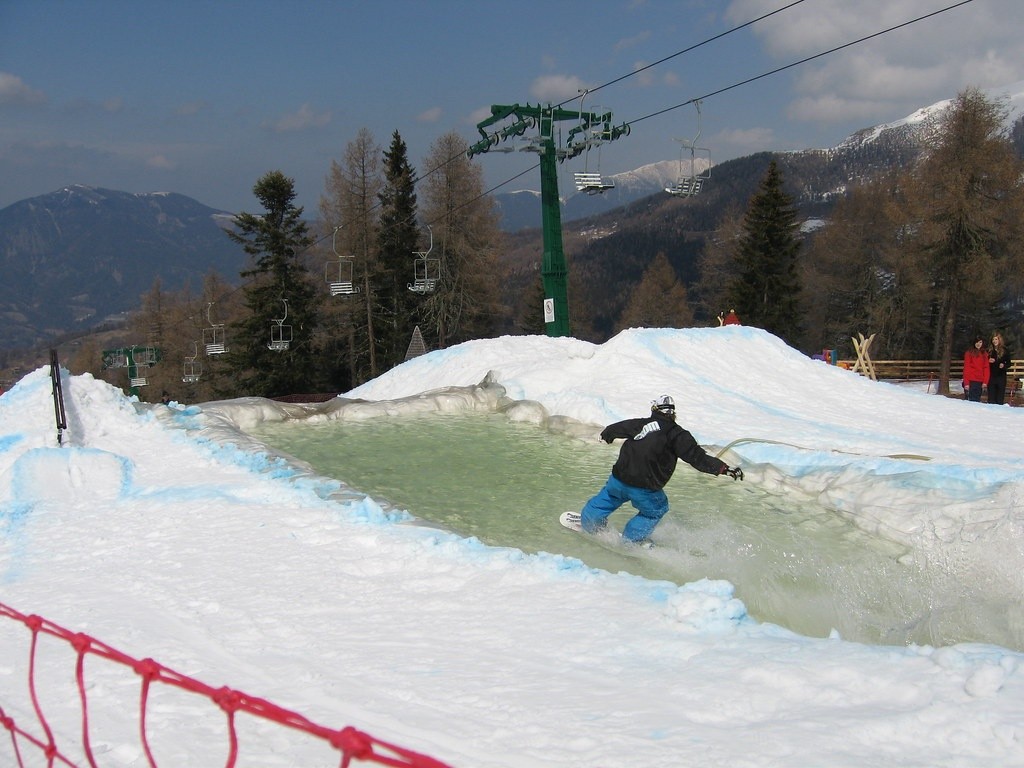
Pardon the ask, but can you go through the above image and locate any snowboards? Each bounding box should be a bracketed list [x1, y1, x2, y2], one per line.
[559, 511, 653, 557]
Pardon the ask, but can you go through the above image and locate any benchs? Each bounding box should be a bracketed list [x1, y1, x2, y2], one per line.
[963, 374, 1022, 401]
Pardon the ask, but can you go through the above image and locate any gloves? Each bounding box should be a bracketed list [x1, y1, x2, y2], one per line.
[722, 465, 744, 481]
[598, 432, 607, 444]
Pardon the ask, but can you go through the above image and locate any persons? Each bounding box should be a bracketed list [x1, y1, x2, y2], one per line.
[581, 395, 745, 549]
[161, 391, 170, 403]
[724, 309, 741, 326]
[963, 333, 1011, 405]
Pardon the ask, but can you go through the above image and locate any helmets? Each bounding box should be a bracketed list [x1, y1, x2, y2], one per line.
[650, 395, 675, 414]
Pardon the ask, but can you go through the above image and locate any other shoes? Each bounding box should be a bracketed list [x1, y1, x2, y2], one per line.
[595, 517, 608, 532]
[634, 538, 653, 550]
